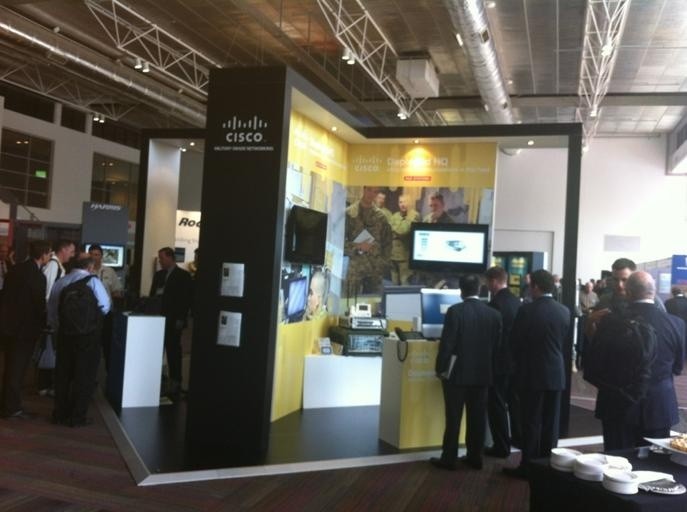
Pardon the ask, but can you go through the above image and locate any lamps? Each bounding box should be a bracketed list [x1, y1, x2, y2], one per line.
[347, 56, 355, 65]
[94, 113, 100, 121]
[100, 115, 105, 123]
[135, 58, 143, 69]
[342, 48, 349, 60]
[143, 63, 150, 72]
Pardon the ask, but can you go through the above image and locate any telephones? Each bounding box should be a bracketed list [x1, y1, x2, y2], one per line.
[349, 303, 372, 318]
[394, 326, 426, 362]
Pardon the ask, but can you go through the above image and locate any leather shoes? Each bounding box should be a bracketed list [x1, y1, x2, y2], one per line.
[457, 456, 482, 469]
[54, 411, 68, 424]
[483, 446, 509, 459]
[510, 436, 522, 448]
[502, 466, 533, 481]
[429, 457, 458, 470]
[50, 415, 70, 424]
[67, 417, 94, 427]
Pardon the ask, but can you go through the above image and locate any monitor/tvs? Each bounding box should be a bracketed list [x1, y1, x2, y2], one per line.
[409, 221, 490, 273]
[283, 276, 309, 324]
[84, 242, 126, 270]
[420, 288, 467, 338]
[380, 285, 426, 318]
[284, 204, 329, 266]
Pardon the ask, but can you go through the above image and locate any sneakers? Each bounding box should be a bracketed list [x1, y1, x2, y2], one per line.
[50, 389, 56, 397]
[8, 407, 24, 416]
[38, 389, 48, 397]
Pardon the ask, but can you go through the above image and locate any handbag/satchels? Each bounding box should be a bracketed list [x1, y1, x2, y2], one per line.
[40, 258, 62, 283]
[31, 334, 57, 369]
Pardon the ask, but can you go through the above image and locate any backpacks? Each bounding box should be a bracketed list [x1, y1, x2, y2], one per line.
[59, 274, 100, 339]
[602, 305, 658, 411]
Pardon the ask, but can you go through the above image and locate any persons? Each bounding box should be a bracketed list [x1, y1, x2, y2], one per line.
[485, 266, 521, 457]
[375, 192, 393, 224]
[183, 248, 199, 276]
[343, 187, 393, 299]
[593, 270, 613, 296]
[664, 284, 687, 376]
[2, 240, 53, 420]
[583, 271, 687, 453]
[303, 272, 328, 321]
[47, 252, 109, 427]
[510, 269, 572, 479]
[33, 240, 77, 396]
[89, 245, 122, 397]
[423, 192, 454, 224]
[390, 194, 422, 286]
[150, 247, 190, 405]
[579, 282, 599, 314]
[431, 274, 502, 471]
[594, 258, 666, 327]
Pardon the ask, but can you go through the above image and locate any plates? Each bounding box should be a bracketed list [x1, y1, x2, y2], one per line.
[585, 452, 632, 473]
[632, 470, 685, 496]
[644, 436, 686, 467]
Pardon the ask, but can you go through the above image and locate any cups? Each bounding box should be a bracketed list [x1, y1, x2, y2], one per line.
[575, 454, 609, 483]
[602, 466, 639, 496]
[550, 448, 584, 473]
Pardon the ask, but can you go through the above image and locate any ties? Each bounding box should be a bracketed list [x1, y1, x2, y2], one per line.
[161, 270, 168, 287]
